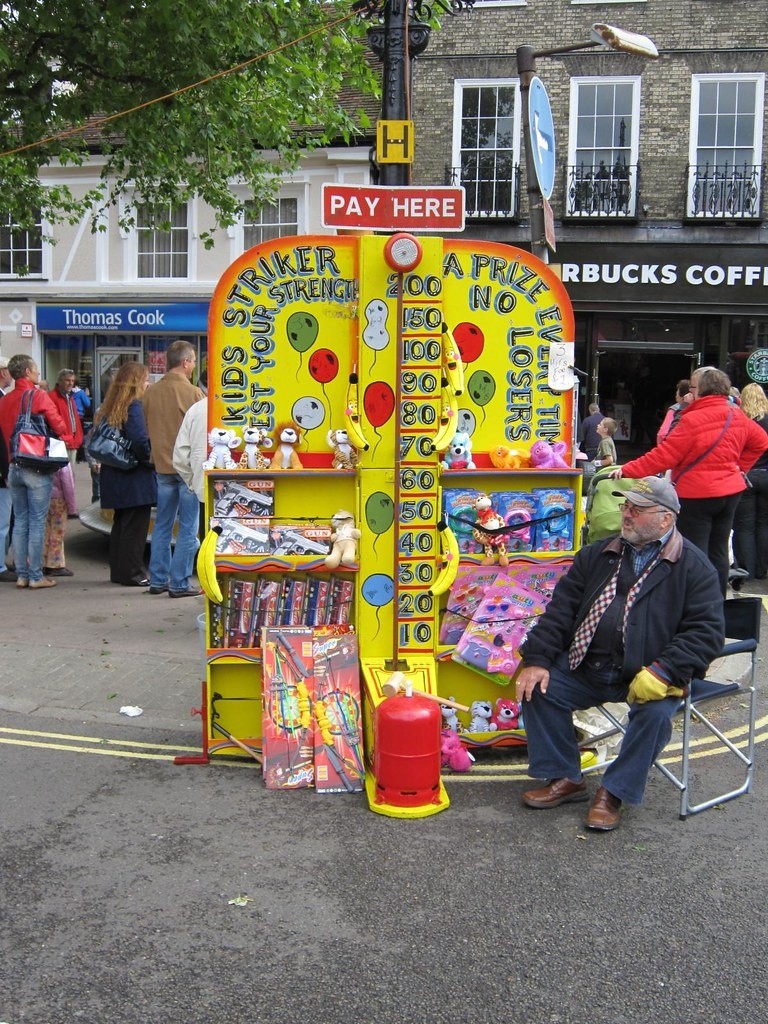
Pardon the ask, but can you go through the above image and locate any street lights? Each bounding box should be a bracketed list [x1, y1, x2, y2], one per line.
[515, 24, 659, 264]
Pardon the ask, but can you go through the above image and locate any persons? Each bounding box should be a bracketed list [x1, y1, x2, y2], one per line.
[0, 341, 207, 598]
[515, 475, 726, 831]
[575, 366, 768, 602]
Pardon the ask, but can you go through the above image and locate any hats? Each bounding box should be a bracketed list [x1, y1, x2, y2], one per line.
[614, 475, 681, 515]
[0, 356, 10, 368]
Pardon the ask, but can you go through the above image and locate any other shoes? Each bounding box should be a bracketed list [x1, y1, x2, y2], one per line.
[17, 576, 28, 587]
[122, 576, 149, 586]
[0, 570, 18, 582]
[29, 576, 55, 588]
[42, 565, 73, 577]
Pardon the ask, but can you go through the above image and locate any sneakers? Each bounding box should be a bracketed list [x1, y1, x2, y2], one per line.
[169, 584, 200, 597]
[150, 583, 170, 593]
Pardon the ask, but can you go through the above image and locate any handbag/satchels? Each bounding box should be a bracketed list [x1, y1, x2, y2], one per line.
[87, 414, 139, 469]
[9, 388, 69, 475]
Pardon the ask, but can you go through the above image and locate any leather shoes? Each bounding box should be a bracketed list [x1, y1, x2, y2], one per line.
[522, 774, 589, 807]
[586, 782, 622, 830]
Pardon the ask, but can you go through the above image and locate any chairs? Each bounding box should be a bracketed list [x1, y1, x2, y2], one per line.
[572, 596, 762, 820]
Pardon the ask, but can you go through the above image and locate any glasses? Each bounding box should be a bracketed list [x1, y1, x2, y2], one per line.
[618, 503, 671, 519]
[688, 384, 697, 391]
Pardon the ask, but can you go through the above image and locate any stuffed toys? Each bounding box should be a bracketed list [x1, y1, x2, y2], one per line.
[439, 730, 475, 772]
[202, 427, 242, 471]
[488, 443, 532, 470]
[471, 494, 509, 567]
[237, 427, 271, 470]
[491, 698, 519, 730]
[324, 508, 362, 569]
[269, 422, 305, 469]
[463, 699, 498, 734]
[327, 428, 364, 471]
[440, 432, 476, 470]
[529, 439, 570, 469]
[516, 702, 525, 730]
[442, 696, 467, 734]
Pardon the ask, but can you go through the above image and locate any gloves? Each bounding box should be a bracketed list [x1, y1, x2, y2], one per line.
[627, 658, 691, 705]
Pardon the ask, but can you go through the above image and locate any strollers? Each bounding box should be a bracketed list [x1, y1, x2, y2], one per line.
[580, 464, 638, 544]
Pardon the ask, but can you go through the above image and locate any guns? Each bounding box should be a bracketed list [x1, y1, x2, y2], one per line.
[216, 518, 270, 553]
[215, 482, 273, 515]
[272, 530, 329, 555]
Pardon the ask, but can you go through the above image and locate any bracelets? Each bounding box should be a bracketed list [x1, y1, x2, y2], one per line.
[594, 460, 602, 466]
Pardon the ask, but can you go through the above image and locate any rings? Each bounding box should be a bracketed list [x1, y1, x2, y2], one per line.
[515, 681, 520, 684]
